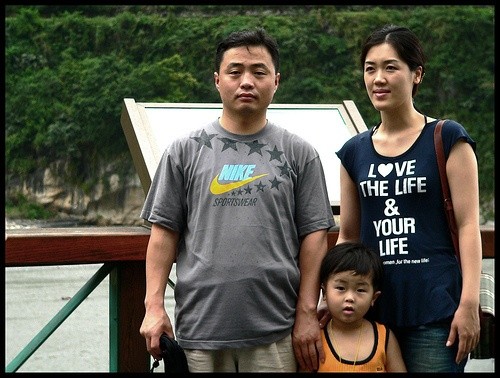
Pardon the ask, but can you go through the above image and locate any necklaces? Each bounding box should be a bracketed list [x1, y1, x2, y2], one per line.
[330, 320, 363, 370]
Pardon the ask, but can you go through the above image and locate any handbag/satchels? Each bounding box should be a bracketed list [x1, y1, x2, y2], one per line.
[469, 272, 495, 359]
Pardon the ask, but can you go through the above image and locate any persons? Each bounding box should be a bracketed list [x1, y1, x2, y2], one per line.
[335, 24, 482, 373]
[320, 241, 408, 373]
[139, 29, 336, 373]
